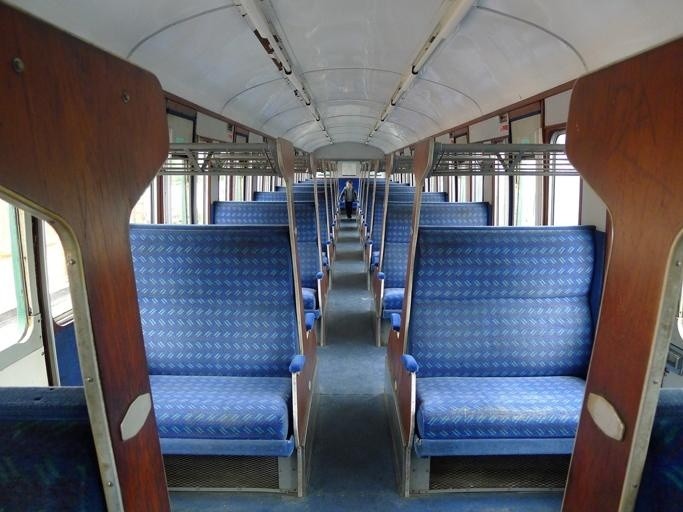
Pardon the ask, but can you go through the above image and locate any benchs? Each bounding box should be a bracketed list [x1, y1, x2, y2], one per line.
[338, 178, 358, 209]
[128, 179, 340, 497]
[355, 179, 596, 496]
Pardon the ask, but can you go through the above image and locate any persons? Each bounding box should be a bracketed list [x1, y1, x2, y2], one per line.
[340, 180, 357, 218]
